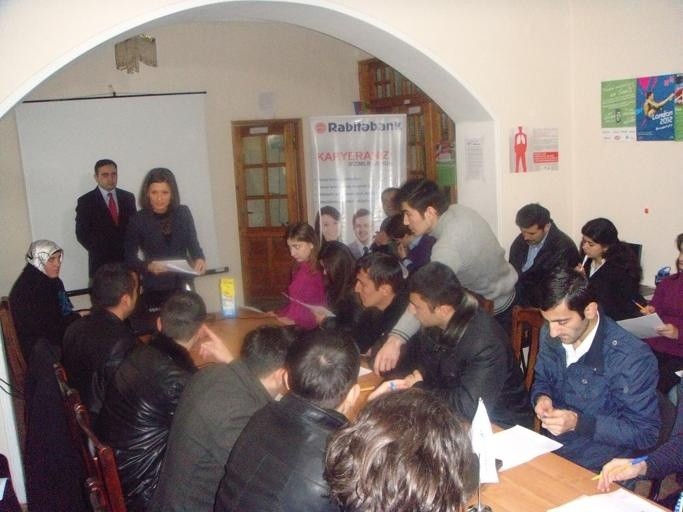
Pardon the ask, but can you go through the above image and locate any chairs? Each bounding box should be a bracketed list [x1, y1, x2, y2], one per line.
[509, 304, 543, 391]
[0, 298, 32, 453]
[71, 403, 130, 511]
[464, 291, 494, 317]
[54, 363, 81, 468]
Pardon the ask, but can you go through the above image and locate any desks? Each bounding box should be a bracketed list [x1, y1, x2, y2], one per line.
[136, 305, 676, 512]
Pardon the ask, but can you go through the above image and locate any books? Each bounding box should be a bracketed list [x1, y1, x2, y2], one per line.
[150, 258, 200, 277]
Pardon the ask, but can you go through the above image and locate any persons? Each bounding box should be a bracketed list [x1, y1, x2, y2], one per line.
[571, 219, 647, 320]
[373, 178, 528, 379]
[75, 160, 138, 276]
[315, 204, 342, 242]
[641, 233, 683, 391]
[389, 215, 436, 269]
[643, 91, 662, 119]
[64, 240, 683, 512]
[506, 201, 578, 306]
[370, 186, 408, 255]
[122, 169, 207, 306]
[8, 237, 78, 363]
[265, 221, 328, 331]
[346, 207, 377, 261]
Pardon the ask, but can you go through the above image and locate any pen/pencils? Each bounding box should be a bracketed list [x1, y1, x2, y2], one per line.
[632, 300, 651, 314]
[360, 385, 376, 392]
[591, 456, 649, 480]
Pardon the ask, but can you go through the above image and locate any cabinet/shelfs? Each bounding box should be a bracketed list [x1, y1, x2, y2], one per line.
[369, 100, 459, 205]
[355, 57, 427, 100]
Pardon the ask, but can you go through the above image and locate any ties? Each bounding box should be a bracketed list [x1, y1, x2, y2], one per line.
[108, 193, 119, 226]
